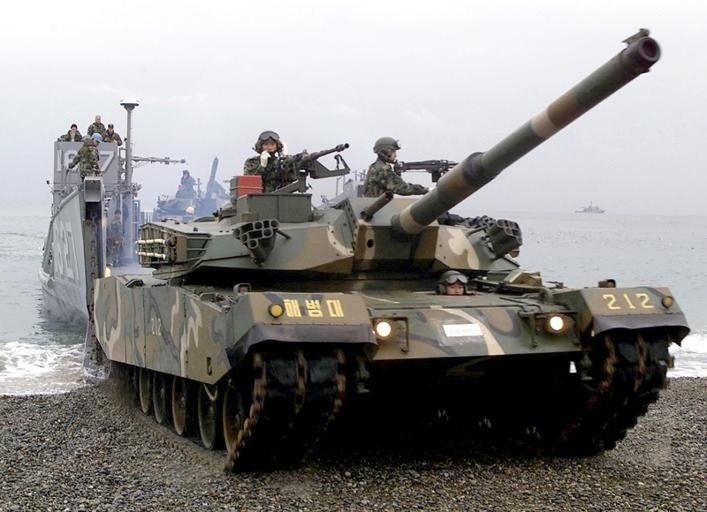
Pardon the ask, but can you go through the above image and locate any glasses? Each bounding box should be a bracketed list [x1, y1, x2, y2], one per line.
[440, 275, 467, 284]
[259, 132, 280, 142]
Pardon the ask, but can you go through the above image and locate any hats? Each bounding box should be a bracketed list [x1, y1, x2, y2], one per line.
[92, 134, 103, 143]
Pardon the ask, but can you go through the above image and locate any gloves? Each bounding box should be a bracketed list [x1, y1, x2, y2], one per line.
[260, 151, 273, 168]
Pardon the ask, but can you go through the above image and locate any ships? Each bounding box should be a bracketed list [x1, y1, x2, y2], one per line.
[38, 98, 186, 329]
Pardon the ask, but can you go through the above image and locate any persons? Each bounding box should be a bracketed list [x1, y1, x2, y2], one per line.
[87, 115, 106, 141]
[109, 209, 125, 266]
[436, 269, 471, 296]
[364, 137, 429, 198]
[61, 124, 82, 141]
[105, 124, 122, 145]
[243, 132, 283, 192]
[179, 170, 197, 193]
[66, 133, 104, 181]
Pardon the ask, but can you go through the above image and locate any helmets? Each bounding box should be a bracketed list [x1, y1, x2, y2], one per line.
[256, 130, 283, 153]
[374, 138, 400, 153]
[438, 271, 468, 294]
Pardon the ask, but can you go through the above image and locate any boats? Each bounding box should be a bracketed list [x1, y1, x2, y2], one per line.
[574, 199, 605, 213]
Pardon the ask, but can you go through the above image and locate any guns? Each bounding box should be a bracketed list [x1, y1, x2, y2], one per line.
[270, 143, 350, 190]
[394, 159, 459, 182]
